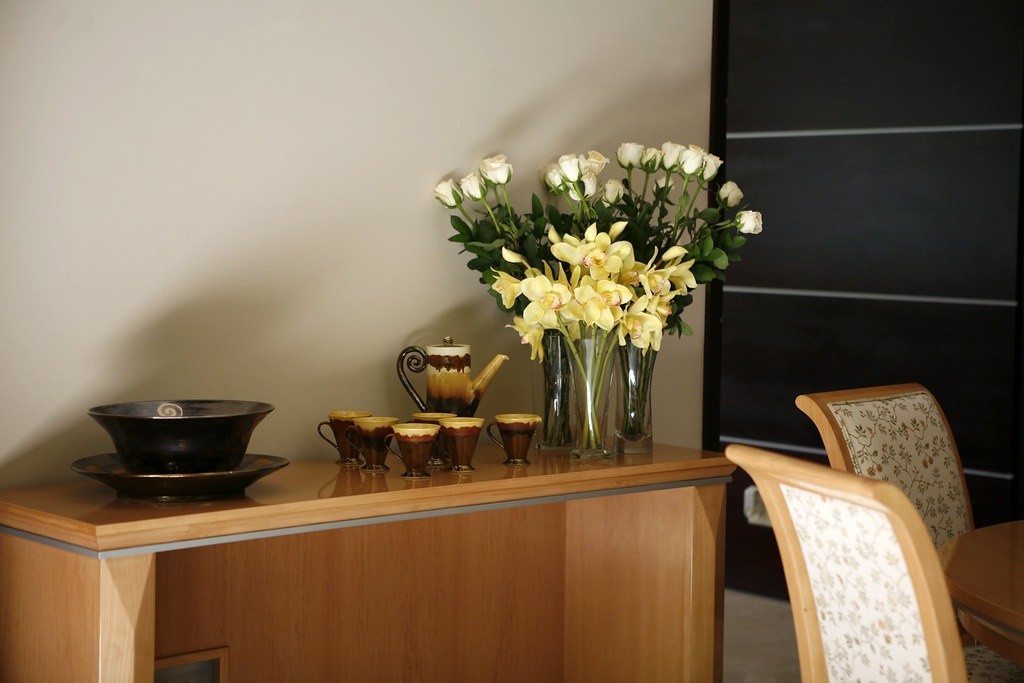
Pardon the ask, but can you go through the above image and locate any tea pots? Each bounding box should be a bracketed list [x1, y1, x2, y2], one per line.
[396, 337, 509, 417]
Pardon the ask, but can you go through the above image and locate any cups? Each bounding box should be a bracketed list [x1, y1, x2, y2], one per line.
[345, 417, 398, 471]
[318, 411, 372, 466]
[487, 414, 542, 465]
[435, 418, 482, 472]
[402, 413, 458, 466]
[385, 423, 441, 478]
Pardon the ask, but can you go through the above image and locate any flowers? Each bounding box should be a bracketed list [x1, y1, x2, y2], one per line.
[430, 138, 768, 449]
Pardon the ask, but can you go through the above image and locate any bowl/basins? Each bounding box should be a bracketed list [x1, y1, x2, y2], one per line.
[87, 400, 275, 473]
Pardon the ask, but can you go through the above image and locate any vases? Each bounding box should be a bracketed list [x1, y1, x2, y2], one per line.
[567, 337, 617, 458]
[530, 333, 578, 454]
[609, 328, 667, 455]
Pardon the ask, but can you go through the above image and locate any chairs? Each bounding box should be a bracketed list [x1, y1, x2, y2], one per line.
[793, 380, 977, 563]
[722, 436, 1012, 683]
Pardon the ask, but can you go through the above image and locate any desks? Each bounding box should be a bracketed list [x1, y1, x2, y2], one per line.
[0, 441, 745, 683]
[933, 517, 1024, 683]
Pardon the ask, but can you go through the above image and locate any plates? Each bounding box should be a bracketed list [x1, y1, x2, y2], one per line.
[73, 452, 289, 503]
